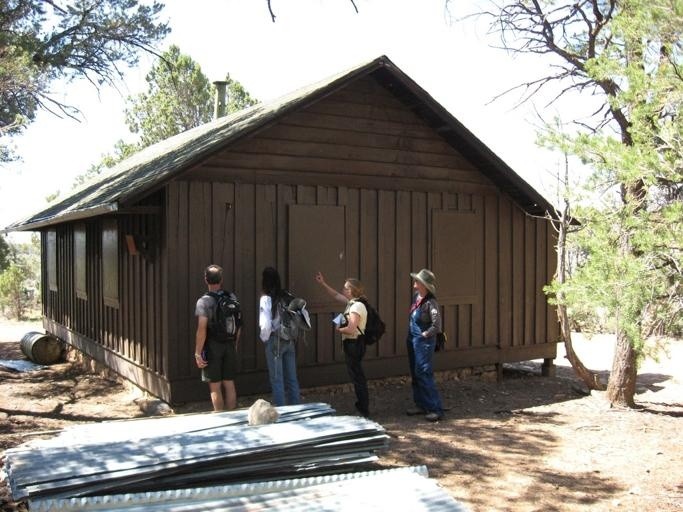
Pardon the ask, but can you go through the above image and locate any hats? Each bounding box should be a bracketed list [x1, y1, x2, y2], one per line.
[409, 268, 436, 294]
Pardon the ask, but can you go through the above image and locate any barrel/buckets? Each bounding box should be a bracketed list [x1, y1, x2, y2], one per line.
[20, 332, 65, 365]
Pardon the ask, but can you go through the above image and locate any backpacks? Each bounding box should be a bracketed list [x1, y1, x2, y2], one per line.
[203, 291, 242, 343]
[273, 291, 312, 341]
[350, 299, 384, 344]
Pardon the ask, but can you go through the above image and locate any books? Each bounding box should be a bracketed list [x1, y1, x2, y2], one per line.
[328, 312, 348, 329]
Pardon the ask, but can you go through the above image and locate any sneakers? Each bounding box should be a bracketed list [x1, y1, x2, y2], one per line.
[406, 406, 426, 416]
[425, 412, 441, 421]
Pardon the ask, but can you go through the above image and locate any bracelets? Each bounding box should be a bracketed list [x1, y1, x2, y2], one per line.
[193, 352, 201, 357]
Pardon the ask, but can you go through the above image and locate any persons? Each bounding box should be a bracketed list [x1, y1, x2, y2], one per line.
[258, 267, 301, 407]
[406, 268, 444, 421]
[192, 264, 245, 411]
[315, 270, 370, 418]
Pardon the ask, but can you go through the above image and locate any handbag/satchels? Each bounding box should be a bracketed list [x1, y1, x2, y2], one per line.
[435, 332, 445, 351]
